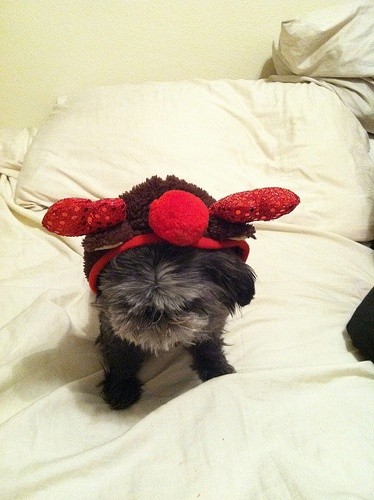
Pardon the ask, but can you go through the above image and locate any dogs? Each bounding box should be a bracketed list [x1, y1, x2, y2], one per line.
[91, 174, 256, 410]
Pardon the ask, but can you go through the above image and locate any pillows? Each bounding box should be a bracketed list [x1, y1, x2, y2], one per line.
[14, 80, 374, 244]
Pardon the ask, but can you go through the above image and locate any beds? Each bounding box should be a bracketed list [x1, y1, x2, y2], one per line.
[0, 129, 374, 500]
